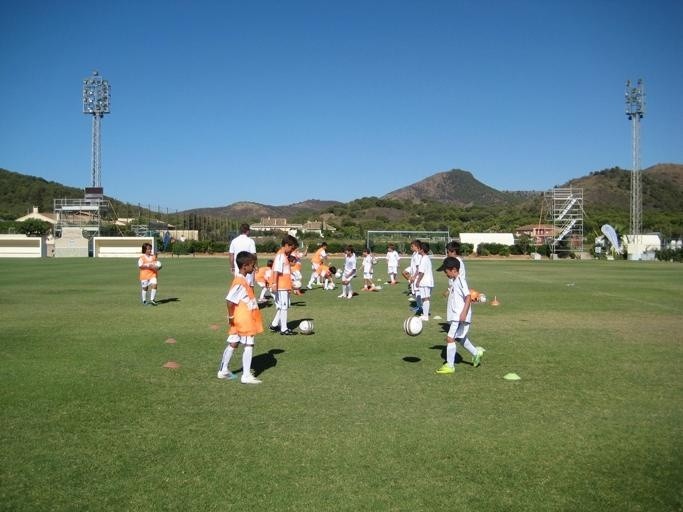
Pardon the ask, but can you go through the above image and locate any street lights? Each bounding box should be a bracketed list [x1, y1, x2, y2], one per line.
[82, 70, 111, 188]
[624, 77, 647, 245]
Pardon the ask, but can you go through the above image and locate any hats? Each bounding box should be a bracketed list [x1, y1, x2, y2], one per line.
[436, 257, 460, 271]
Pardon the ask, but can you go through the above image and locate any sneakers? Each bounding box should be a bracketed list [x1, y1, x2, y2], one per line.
[240, 373, 263, 383]
[433, 363, 456, 374]
[258, 297, 268, 302]
[149, 300, 158, 306]
[268, 323, 280, 331]
[471, 346, 486, 368]
[280, 329, 297, 335]
[217, 370, 238, 380]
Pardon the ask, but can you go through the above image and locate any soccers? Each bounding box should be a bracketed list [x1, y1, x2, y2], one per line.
[338, 269, 343, 273]
[335, 272, 340, 277]
[327, 282, 335, 291]
[292, 280, 301, 288]
[152, 261, 161, 270]
[476, 294, 486, 303]
[299, 321, 314, 335]
[403, 317, 422, 336]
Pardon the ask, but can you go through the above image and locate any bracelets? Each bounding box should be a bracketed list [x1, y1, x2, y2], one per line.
[228, 316, 234, 319]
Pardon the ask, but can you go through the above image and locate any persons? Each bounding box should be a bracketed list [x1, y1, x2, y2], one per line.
[306, 242, 336, 290]
[256, 248, 302, 302]
[437, 241, 484, 374]
[229, 224, 258, 294]
[269, 235, 299, 335]
[138, 242, 158, 305]
[339, 245, 357, 300]
[385, 243, 400, 285]
[359, 247, 376, 291]
[217, 251, 265, 383]
[403, 240, 435, 321]
